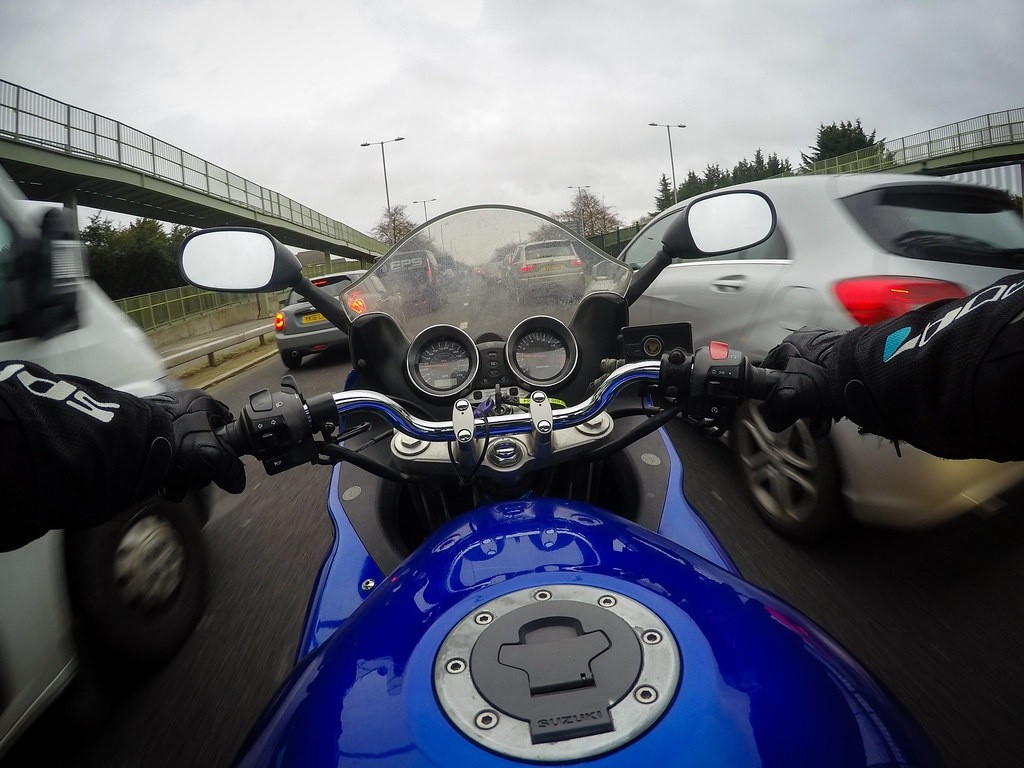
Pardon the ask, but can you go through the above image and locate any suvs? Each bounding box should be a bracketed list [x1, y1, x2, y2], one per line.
[506, 238, 586, 307]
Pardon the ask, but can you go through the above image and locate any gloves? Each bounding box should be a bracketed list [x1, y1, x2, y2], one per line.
[139, 388, 246, 496]
[752, 327, 851, 440]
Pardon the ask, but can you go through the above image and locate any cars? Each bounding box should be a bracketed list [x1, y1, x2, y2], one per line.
[438, 245, 523, 293]
[579, 176, 1020, 547]
[272, 270, 401, 370]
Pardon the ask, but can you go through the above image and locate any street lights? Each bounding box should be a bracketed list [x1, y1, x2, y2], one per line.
[411, 199, 438, 242]
[648, 121, 687, 205]
[361, 136, 405, 245]
[568, 185, 591, 239]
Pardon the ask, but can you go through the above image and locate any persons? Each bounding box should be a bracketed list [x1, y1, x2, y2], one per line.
[0, 271, 1024, 558]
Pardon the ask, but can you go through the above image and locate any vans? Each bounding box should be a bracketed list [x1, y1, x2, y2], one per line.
[0, 164, 214, 754]
[372, 250, 448, 318]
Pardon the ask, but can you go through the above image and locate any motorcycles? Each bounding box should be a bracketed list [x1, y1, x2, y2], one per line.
[0, 189, 1022, 768]
